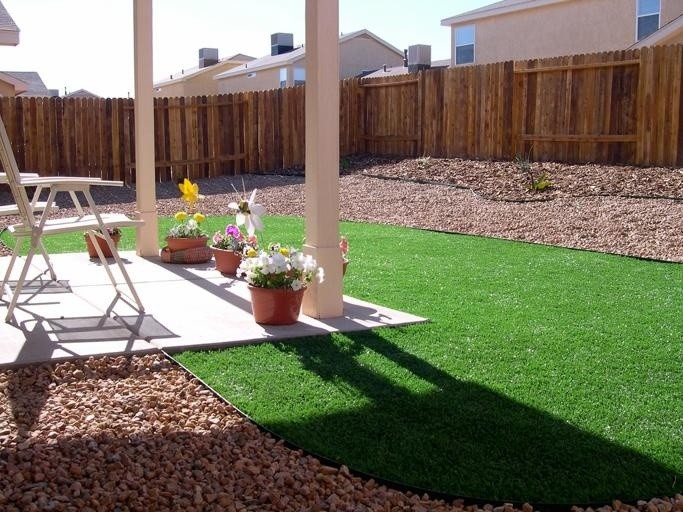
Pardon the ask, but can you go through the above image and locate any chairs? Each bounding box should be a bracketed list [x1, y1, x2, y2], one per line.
[1, 114, 146, 324]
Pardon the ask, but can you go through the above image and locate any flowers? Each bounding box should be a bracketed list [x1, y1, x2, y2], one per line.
[340, 235, 348, 259]
[235, 242, 326, 290]
[212, 177, 264, 251]
[167, 178, 209, 237]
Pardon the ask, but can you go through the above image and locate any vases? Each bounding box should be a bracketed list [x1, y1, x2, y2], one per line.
[165, 235, 207, 251]
[343, 259, 350, 276]
[208, 245, 241, 276]
[247, 284, 310, 325]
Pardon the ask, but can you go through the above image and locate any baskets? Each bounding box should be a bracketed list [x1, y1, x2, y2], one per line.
[161, 245, 214, 264]
